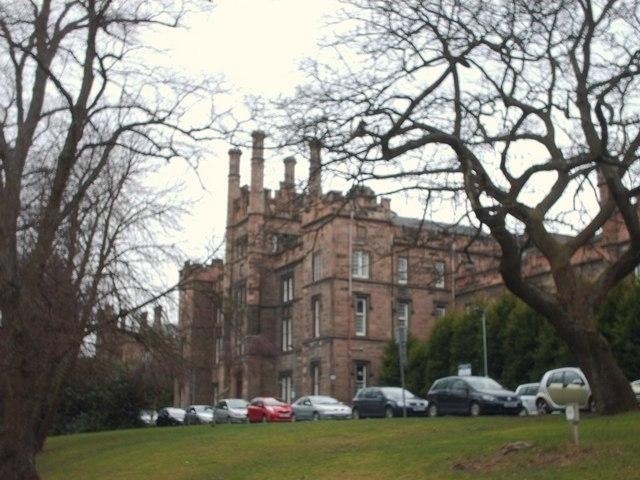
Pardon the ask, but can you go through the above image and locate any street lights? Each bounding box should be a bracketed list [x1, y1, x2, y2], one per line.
[393, 316, 409, 419]
[476, 305, 489, 378]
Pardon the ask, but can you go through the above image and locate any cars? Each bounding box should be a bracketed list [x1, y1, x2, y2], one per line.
[425, 374, 523, 418]
[288, 394, 352, 422]
[212, 397, 251, 424]
[514, 381, 559, 415]
[534, 364, 598, 414]
[183, 404, 214, 425]
[140, 410, 159, 426]
[156, 406, 187, 427]
[246, 395, 292, 421]
[350, 385, 428, 420]
